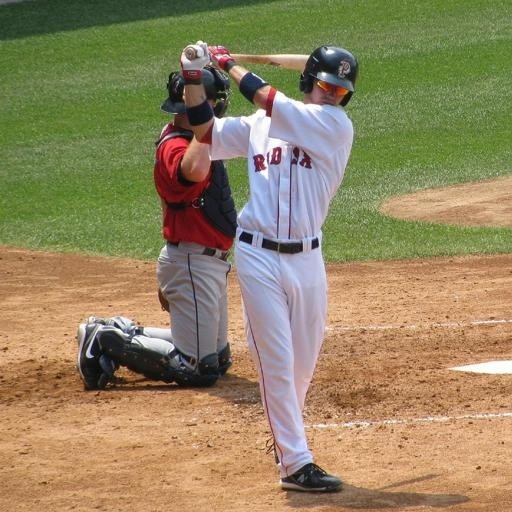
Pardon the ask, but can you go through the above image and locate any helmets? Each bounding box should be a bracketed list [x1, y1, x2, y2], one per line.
[299, 46, 358, 106]
[160, 63, 230, 117]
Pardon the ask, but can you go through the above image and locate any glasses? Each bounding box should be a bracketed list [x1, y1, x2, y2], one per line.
[316, 79, 349, 97]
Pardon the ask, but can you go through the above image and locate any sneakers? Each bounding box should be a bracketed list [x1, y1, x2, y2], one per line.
[279, 464, 344, 492]
[78, 314, 115, 389]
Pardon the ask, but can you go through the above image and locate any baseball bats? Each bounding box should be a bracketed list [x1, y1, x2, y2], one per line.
[184, 46, 311, 72]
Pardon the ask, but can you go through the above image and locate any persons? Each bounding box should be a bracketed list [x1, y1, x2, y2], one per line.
[76, 65, 237, 390]
[176, 39, 360, 491]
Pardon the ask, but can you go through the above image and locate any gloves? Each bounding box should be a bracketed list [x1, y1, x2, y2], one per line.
[209, 45, 237, 72]
[180, 39, 210, 84]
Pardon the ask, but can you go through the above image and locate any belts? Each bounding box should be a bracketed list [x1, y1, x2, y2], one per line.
[238, 231, 319, 253]
[166, 238, 230, 261]
